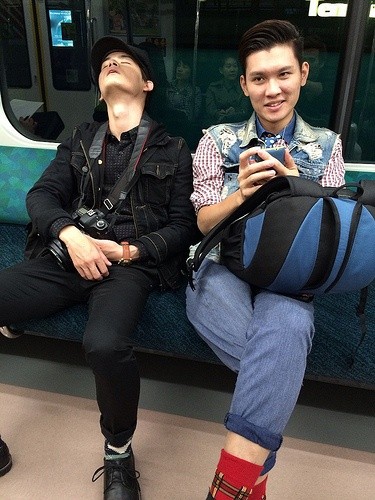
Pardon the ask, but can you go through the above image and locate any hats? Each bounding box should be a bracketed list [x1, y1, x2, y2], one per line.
[90, 36, 157, 95]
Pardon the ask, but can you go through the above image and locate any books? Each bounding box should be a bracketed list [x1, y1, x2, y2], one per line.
[9, 99, 45, 122]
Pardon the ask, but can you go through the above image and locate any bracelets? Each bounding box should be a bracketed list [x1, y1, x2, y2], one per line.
[306, 81, 312, 90]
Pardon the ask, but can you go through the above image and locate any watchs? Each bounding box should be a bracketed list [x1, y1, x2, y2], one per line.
[117, 242, 133, 267]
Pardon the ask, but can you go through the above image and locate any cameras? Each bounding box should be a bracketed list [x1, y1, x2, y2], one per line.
[47, 205, 116, 272]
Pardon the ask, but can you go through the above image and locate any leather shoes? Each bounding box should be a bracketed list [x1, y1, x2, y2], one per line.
[92, 440, 143, 499]
[0, 435, 12, 477]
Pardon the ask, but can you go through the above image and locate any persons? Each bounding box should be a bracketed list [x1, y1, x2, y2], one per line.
[300, 34, 334, 128]
[186, 19, 345, 500]
[205, 56, 250, 124]
[0, 35, 205, 500]
[147, 38, 166, 56]
[165, 56, 201, 139]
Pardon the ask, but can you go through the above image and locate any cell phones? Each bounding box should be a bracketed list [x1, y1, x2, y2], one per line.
[253, 147, 286, 186]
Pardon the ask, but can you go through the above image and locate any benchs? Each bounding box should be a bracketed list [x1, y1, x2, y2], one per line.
[0, 222, 375, 393]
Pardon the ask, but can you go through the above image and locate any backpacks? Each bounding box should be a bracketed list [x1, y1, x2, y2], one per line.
[183, 175, 375, 373]
